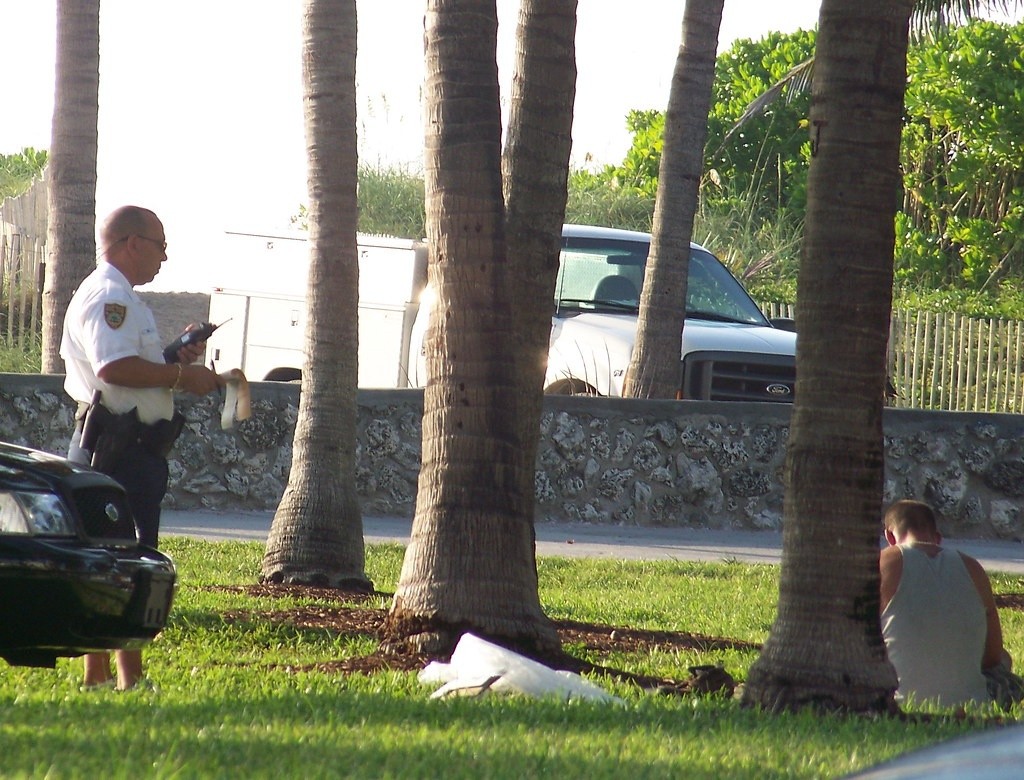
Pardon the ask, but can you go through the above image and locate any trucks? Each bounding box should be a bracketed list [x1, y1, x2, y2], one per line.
[189, 210, 797, 405]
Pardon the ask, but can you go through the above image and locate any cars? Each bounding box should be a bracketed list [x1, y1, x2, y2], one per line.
[1, 440, 178, 669]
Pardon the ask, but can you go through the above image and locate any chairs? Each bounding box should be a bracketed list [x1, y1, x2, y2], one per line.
[592, 274, 640, 310]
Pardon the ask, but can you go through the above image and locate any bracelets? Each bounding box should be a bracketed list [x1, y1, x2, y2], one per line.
[170, 363, 183, 392]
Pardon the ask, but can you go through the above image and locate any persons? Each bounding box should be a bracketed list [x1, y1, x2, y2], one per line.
[59, 205, 226, 691]
[879, 500, 1024, 718]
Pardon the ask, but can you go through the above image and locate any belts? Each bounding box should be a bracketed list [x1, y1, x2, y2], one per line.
[101, 414, 152, 445]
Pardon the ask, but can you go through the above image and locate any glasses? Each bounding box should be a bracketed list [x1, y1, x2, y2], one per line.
[116, 235, 167, 252]
